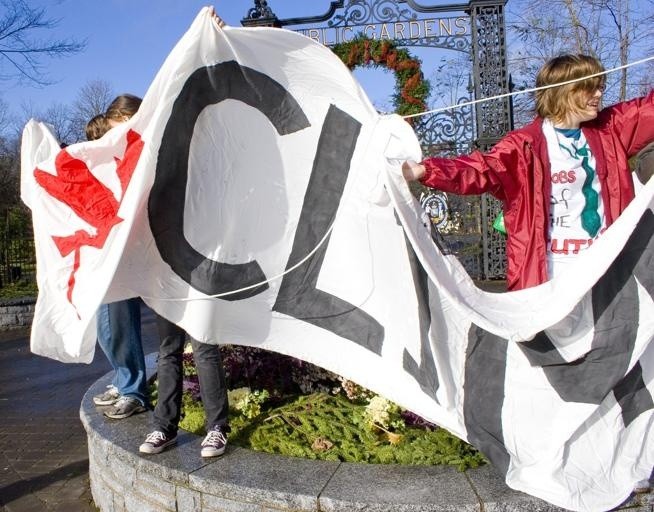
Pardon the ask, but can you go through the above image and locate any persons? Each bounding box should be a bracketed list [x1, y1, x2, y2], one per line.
[85, 113, 148, 419]
[402, 54, 654, 292]
[104, 6, 232, 459]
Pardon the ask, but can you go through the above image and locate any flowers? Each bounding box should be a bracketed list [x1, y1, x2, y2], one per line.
[334, 34, 430, 128]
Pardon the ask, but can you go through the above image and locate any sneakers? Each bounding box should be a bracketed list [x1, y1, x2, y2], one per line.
[92, 384, 147, 419]
[139, 430, 178, 454]
[200, 424, 229, 458]
